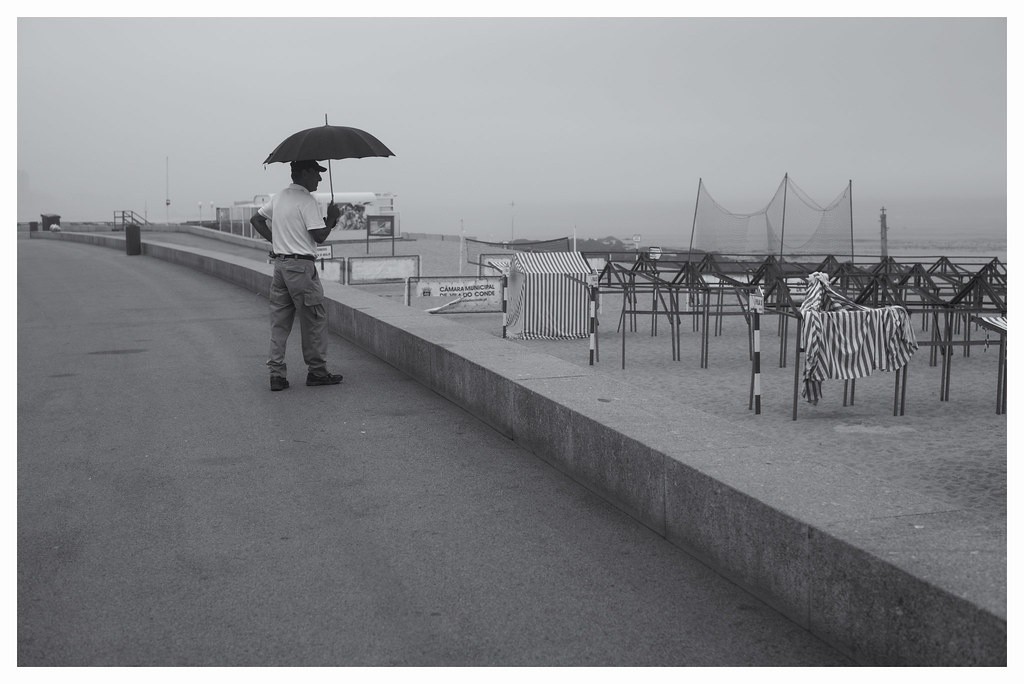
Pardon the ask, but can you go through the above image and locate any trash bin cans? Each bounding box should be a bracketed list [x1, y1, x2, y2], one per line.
[125, 223, 141, 256]
[40, 213, 62, 231]
[28, 221, 39, 239]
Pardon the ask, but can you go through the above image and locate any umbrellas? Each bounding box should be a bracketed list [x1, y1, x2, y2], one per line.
[262, 113, 396, 228]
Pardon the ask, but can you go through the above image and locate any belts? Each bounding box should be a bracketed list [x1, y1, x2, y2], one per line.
[274, 253, 316, 262]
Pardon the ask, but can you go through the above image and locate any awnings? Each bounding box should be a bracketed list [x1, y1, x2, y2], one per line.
[488, 251, 1007, 421]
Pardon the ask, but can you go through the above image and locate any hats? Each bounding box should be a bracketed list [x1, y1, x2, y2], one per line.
[290, 160, 327, 172]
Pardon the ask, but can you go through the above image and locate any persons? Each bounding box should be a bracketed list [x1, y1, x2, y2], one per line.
[250, 160, 343, 391]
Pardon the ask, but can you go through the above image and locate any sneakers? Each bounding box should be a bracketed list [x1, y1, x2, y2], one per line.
[270, 377, 289, 391]
[306, 373, 343, 386]
